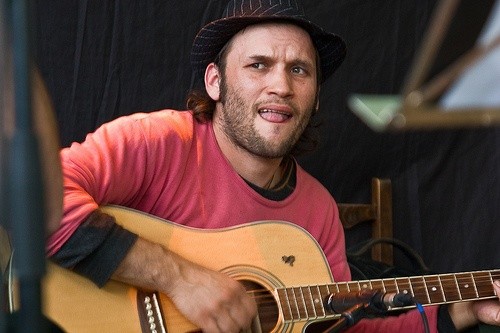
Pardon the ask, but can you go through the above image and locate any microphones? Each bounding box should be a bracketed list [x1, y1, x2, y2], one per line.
[324, 290, 413, 315]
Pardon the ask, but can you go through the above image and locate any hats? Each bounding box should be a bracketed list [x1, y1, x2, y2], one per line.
[190, 0, 348, 86]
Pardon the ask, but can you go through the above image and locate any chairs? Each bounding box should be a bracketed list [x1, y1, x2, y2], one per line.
[338, 177, 394, 266]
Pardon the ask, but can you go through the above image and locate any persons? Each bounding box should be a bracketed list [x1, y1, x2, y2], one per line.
[45, 0, 500, 333]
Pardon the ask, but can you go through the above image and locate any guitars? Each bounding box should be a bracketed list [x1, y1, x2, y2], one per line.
[8, 204, 500, 333]
